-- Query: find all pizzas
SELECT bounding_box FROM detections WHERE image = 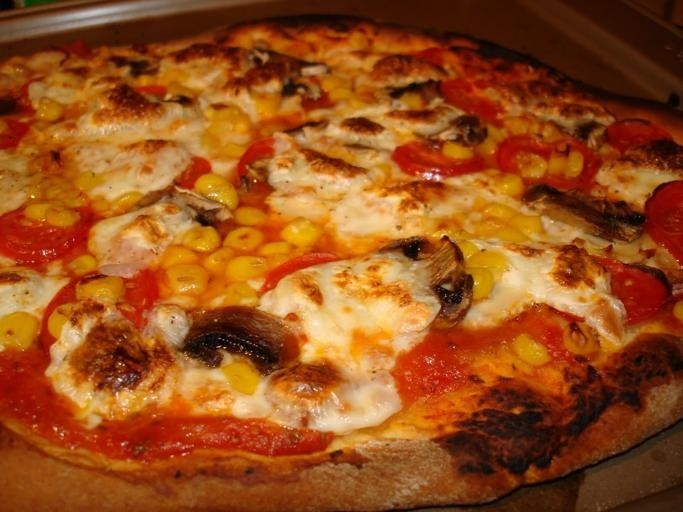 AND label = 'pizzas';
[0,12,683,512]
